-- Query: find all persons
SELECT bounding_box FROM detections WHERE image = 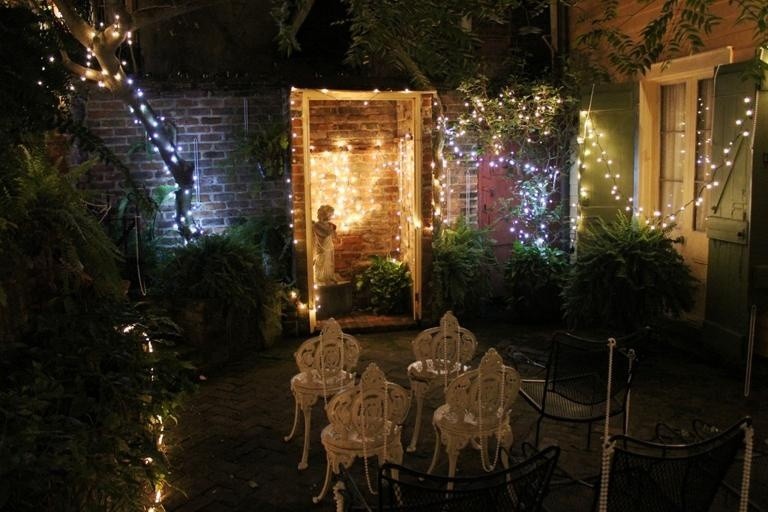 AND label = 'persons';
[312,205,340,283]
